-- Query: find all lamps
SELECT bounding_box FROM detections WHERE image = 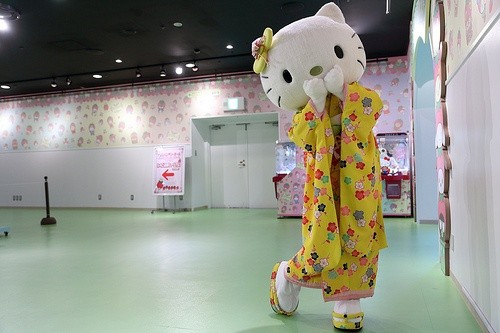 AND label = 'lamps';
[192,60,198,72]
[159,64,167,77]
[135,67,142,78]
